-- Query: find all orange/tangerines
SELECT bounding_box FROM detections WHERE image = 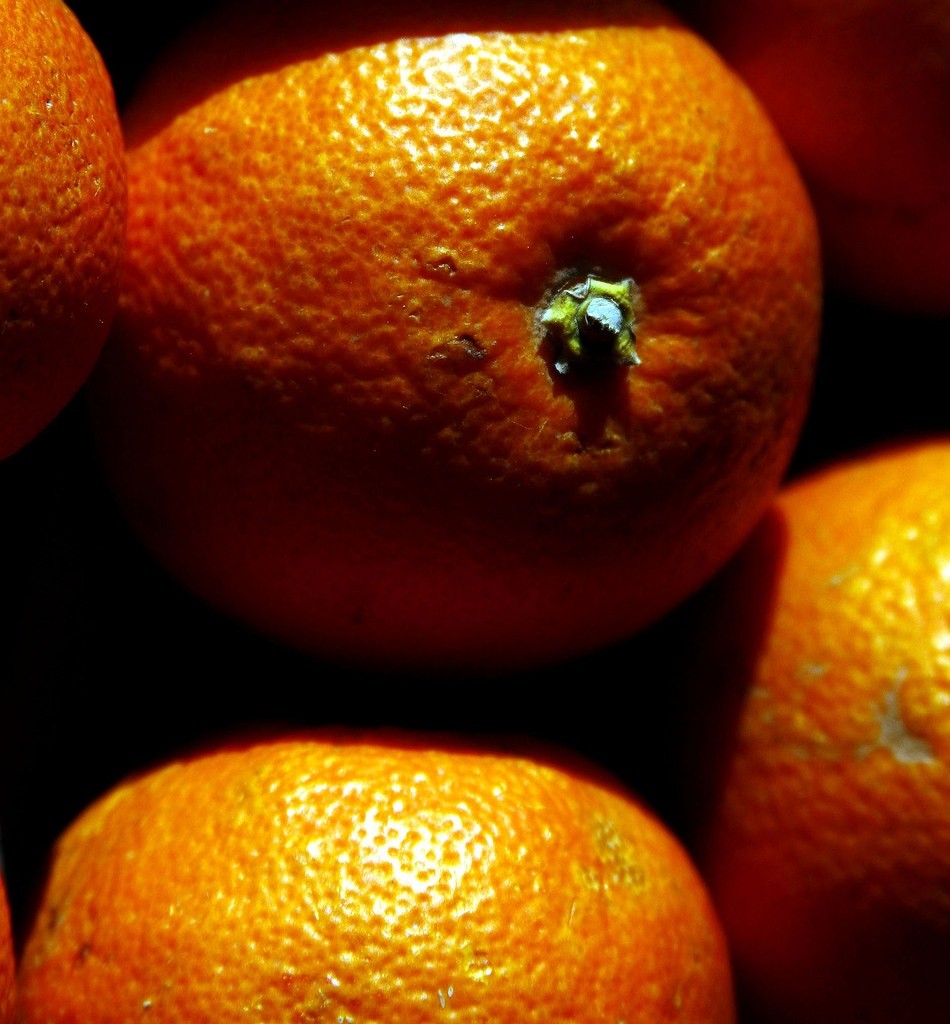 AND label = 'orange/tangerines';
[0,0,950,1024]
